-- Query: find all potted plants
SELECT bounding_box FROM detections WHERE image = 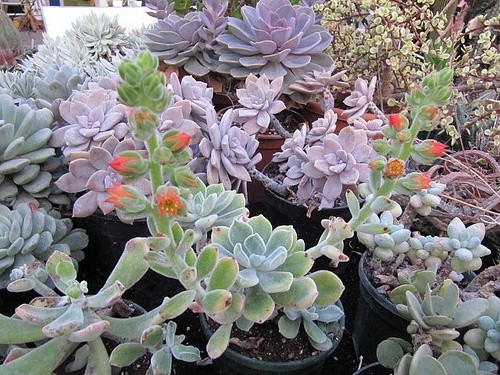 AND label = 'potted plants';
[0,0,499,375]
